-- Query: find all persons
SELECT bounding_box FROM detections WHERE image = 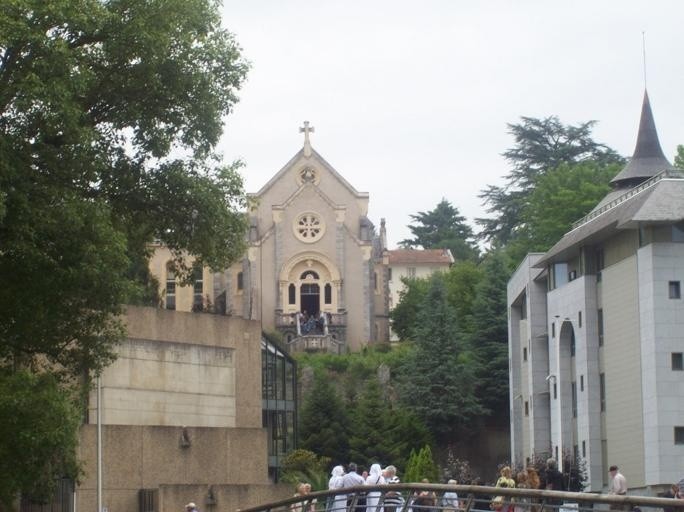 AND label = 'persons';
[185,502,200,512]
[288,460,570,512]
[607,464,634,511]
[661,478,683,511]
